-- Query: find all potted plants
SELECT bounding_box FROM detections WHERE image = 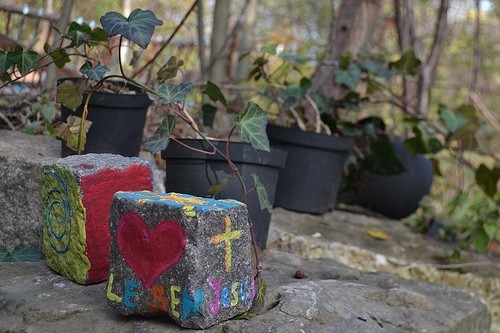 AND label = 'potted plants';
[238,43,391,217]
[338,48,479,223]
[0,7,164,159]
[142,78,290,251]
